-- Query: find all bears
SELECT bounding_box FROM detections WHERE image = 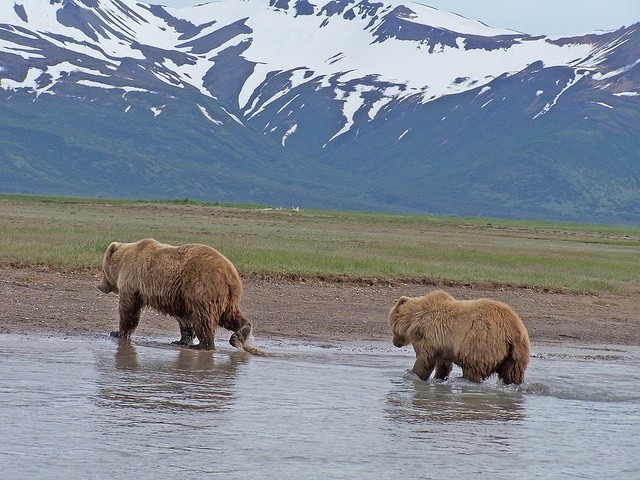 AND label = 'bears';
[97,238,252,351]
[389,289,532,387]
